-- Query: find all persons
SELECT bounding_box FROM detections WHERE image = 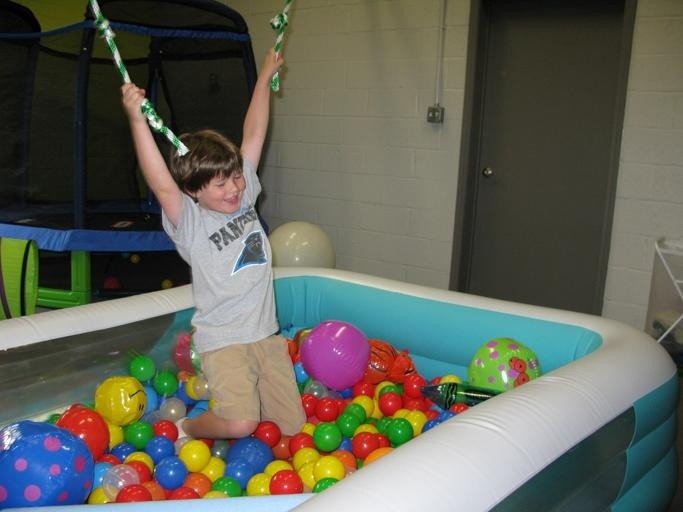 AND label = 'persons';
[120,46,306,435]
